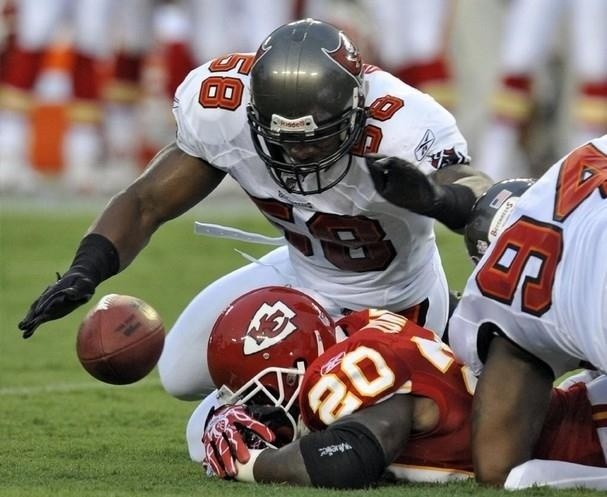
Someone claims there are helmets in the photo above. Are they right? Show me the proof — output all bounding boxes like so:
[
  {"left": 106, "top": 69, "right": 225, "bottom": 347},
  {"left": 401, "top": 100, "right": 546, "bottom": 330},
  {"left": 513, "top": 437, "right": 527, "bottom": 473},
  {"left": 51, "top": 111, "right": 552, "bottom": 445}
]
[
  {"left": 207, "top": 286, "right": 336, "bottom": 410},
  {"left": 246, "top": 18, "right": 367, "bottom": 144},
  {"left": 464, "top": 178, "right": 535, "bottom": 265}
]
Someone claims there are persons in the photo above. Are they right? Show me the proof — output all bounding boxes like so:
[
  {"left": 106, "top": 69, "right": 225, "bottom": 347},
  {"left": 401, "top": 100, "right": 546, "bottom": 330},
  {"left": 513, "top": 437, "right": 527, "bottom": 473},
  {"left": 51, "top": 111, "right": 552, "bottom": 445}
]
[
  {"left": 18, "top": 17, "right": 492, "bottom": 401},
  {"left": 202, "top": 286, "right": 606, "bottom": 486},
  {"left": 448, "top": 133, "right": 606, "bottom": 492}
]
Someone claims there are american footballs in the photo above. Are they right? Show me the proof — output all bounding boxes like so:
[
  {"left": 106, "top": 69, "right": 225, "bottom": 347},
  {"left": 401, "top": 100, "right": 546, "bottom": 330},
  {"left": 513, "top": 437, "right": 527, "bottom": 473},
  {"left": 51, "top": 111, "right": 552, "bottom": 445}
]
[{"left": 77, "top": 294, "right": 166, "bottom": 385}]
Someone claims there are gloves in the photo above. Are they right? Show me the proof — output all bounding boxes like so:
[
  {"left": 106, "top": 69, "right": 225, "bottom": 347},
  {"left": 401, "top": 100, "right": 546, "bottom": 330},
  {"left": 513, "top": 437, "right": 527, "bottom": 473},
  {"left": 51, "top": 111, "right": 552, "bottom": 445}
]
[
  {"left": 201, "top": 403, "right": 276, "bottom": 483},
  {"left": 365, "top": 153, "right": 457, "bottom": 222},
  {"left": 17, "top": 234, "right": 119, "bottom": 340}
]
